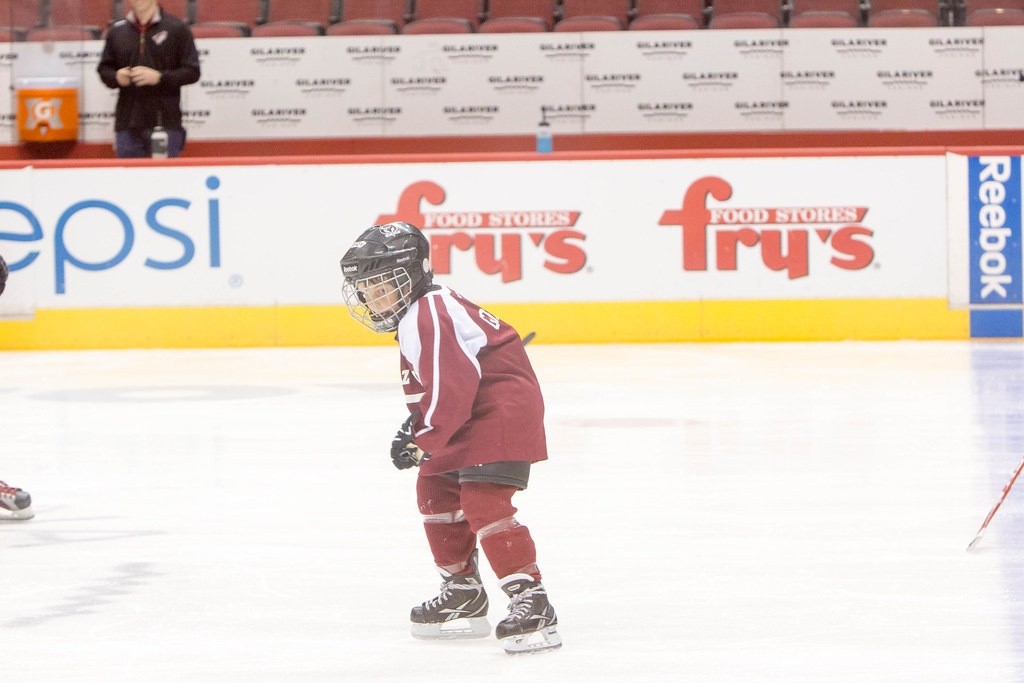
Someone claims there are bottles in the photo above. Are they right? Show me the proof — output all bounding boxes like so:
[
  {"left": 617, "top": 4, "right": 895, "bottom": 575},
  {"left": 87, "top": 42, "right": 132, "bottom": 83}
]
[
  {"left": 536, "top": 121, "right": 552, "bottom": 152},
  {"left": 150, "top": 126, "right": 169, "bottom": 159}
]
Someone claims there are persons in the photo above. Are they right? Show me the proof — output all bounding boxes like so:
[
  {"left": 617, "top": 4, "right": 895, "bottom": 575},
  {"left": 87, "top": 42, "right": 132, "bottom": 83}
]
[
  {"left": 339, "top": 219, "right": 561, "bottom": 652},
  {"left": 0, "top": 478, "right": 35, "bottom": 520},
  {"left": 96, "top": 1, "right": 201, "bottom": 158}
]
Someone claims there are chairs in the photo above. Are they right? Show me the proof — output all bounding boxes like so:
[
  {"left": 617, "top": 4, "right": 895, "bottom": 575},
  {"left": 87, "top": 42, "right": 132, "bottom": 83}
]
[{"left": 0, "top": 0, "right": 1023, "bottom": 42}]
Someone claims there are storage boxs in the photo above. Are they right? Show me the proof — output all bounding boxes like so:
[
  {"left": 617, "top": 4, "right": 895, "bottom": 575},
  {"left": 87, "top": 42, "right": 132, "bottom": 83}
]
[{"left": 15, "top": 88, "right": 79, "bottom": 142}]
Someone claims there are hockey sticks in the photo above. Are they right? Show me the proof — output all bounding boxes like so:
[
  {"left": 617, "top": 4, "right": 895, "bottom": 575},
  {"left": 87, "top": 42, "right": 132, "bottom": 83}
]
[
  {"left": 400, "top": 332, "right": 535, "bottom": 459},
  {"left": 965, "top": 454, "right": 1024, "bottom": 554}
]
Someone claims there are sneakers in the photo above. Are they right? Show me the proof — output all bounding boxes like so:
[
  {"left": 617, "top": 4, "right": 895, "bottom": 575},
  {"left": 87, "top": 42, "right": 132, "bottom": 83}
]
[
  {"left": 495, "top": 573, "right": 562, "bottom": 657},
  {"left": 409, "top": 548, "right": 492, "bottom": 640}
]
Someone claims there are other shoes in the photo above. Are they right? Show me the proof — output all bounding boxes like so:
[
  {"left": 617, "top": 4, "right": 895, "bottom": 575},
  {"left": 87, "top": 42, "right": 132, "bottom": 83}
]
[{"left": 0, "top": 481, "right": 35, "bottom": 521}]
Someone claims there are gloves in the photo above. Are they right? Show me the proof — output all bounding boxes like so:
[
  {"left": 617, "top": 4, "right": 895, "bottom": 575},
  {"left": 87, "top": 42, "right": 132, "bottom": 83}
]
[{"left": 390, "top": 416, "right": 432, "bottom": 470}]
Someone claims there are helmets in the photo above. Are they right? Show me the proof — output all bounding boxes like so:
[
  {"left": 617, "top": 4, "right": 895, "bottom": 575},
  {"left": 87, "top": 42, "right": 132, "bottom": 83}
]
[{"left": 340, "top": 221, "right": 433, "bottom": 333}]
